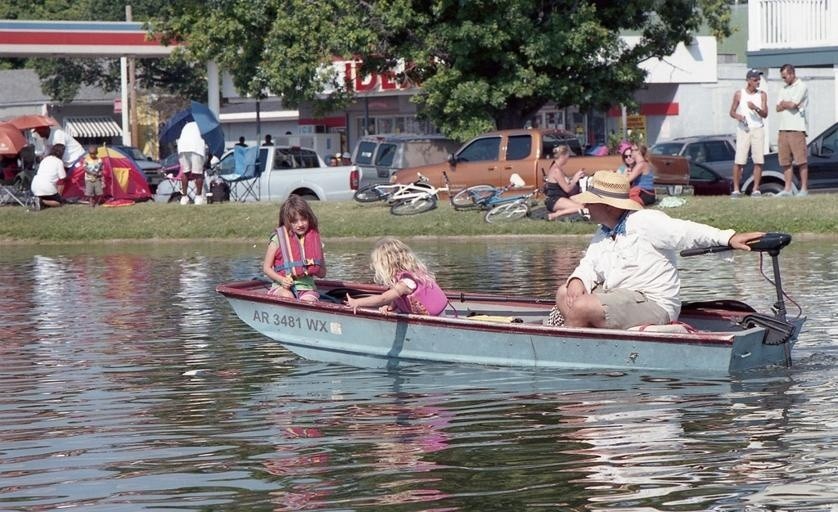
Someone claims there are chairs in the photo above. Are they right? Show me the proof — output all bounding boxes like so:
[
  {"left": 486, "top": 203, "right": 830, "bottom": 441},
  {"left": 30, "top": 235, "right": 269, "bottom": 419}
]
[
  {"left": 218, "top": 144, "right": 264, "bottom": 204},
  {"left": 159, "top": 163, "right": 197, "bottom": 199}
]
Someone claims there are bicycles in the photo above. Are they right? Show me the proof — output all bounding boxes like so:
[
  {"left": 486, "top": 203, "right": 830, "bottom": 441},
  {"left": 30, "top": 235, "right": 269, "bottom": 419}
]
[
  {"left": 352, "top": 170, "right": 454, "bottom": 216},
  {"left": 452, "top": 168, "right": 551, "bottom": 225}
]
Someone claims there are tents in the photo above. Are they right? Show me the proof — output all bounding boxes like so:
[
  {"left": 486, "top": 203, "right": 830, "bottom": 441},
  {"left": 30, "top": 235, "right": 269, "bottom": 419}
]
[{"left": 61, "top": 142, "right": 154, "bottom": 206}]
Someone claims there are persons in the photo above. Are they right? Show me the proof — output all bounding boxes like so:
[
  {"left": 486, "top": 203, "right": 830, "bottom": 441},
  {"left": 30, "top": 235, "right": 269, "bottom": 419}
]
[
  {"left": 555, "top": 169, "right": 767, "bottom": 328},
  {"left": 176, "top": 121, "right": 208, "bottom": 206},
  {"left": 728, "top": 68, "right": 768, "bottom": 198},
  {"left": 625, "top": 141, "right": 656, "bottom": 206},
  {"left": 82, "top": 145, "right": 106, "bottom": 208},
  {"left": 542, "top": 145, "right": 587, "bottom": 220},
  {"left": 341, "top": 236, "right": 450, "bottom": 318},
  {"left": 615, "top": 147, "right": 636, "bottom": 177},
  {"left": 262, "top": 194, "right": 327, "bottom": 302},
  {"left": 774, "top": 63, "right": 809, "bottom": 198},
  {"left": 234, "top": 136, "right": 248, "bottom": 147},
  {"left": 328, "top": 152, "right": 353, "bottom": 166},
  {"left": 261, "top": 134, "right": 274, "bottom": 146},
  {"left": 0, "top": 120, "right": 84, "bottom": 211}
]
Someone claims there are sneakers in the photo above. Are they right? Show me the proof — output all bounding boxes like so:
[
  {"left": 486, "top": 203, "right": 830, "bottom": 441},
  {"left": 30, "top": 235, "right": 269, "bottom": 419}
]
[
  {"left": 34, "top": 197, "right": 41, "bottom": 211},
  {"left": 180, "top": 196, "right": 189, "bottom": 205},
  {"left": 797, "top": 190, "right": 807, "bottom": 198},
  {"left": 731, "top": 192, "right": 741, "bottom": 199},
  {"left": 751, "top": 190, "right": 761, "bottom": 198},
  {"left": 194, "top": 195, "right": 203, "bottom": 205},
  {"left": 775, "top": 190, "right": 793, "bottom": 196}
]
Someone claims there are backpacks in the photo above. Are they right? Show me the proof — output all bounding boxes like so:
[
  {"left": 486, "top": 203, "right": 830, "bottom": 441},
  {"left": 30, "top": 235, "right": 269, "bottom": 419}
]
[{"left": 209, "top": 179, "right": 229, "bottom": 203}]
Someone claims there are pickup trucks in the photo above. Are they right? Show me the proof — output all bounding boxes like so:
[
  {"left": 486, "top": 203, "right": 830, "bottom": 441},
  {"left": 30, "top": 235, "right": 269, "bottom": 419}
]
[
  {"left": 646, "top": 134, "right": 735, "bottom": 179},
  {"left": 388, "top": 127, "right": 690, "bottom": 201},
  {"left": 155, "top": 146, "right": 363, "bottom": 202}
]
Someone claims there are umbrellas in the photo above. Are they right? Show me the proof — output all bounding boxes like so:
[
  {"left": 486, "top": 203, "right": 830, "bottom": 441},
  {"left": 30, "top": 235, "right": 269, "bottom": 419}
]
[
  {"left": 157, "top": 108, "right": 195, "bottom": 145},
  {"left": 6, "top": 114, "right": 56, "bottom": 129},
  {"left": 190, "top": 99, "right": 224, "bottom": 159}
]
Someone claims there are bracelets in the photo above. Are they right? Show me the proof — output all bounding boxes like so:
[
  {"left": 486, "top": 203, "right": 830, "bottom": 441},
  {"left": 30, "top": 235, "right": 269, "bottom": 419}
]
[{"left": 755, "top": 107, "right": 761, "bottom": 113}]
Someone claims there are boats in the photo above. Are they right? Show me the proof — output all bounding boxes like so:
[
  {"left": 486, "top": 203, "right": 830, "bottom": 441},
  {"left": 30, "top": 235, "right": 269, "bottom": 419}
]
[{"left": 216, "top": 232, "right": 808, "bottom": 377}]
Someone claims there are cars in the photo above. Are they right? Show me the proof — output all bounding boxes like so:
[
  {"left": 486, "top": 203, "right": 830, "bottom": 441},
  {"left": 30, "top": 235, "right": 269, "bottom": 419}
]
[
  {"left": 112, "top": 145, "right": 164, "bottom": 185},
  {"left": 740, "top": 121, "right": 838, "bottom": 195}
]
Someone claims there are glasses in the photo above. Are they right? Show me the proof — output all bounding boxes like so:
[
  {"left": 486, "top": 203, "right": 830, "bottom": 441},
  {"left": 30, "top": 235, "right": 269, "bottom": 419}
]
[{"left": 624, "top": 155, "right": 630, "bottom": 158}]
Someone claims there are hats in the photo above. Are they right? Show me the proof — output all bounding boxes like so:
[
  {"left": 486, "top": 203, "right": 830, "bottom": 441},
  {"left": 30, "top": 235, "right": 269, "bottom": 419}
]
[
  {"left": 569, "top": 170, "right": 644, "bottom": 210},
  {"left": 746, "top": 69, "right": 763, "bottom": 78}
]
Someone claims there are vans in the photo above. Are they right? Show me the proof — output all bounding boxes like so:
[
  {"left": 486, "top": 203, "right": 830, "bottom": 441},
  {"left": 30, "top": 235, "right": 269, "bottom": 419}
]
[{"left": 351, "top": 134, "right": 460, "bottom": 185}]
